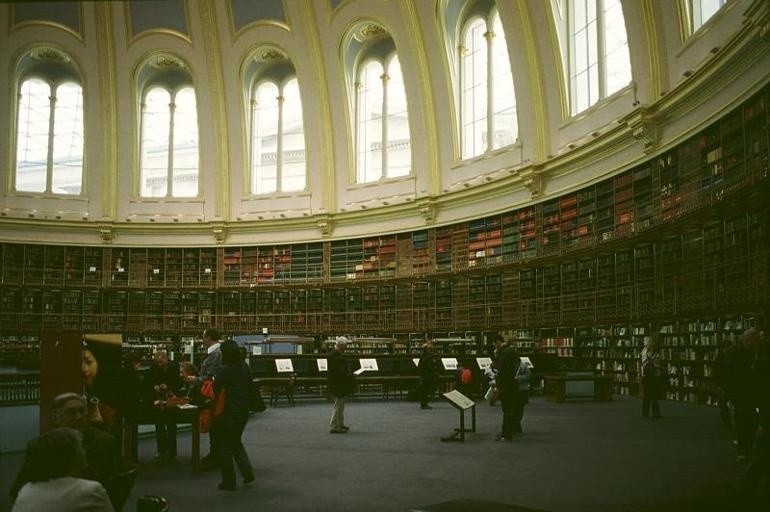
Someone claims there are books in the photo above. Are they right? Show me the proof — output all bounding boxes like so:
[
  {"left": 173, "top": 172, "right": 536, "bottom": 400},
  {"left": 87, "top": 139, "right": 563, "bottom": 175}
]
[
  {"left": 330, "top": 229, "right": 429, "bottom": 356},
  {"left": 660, "top": 91, "right": 769, "bottom": 407},
  {"left": 3, "top": 243, "right": 102, "bottom": 355},
  {"left": 109, "top": 247, "right": 216, "bottom": 368},
  {"left": 561, "top": 160, "right": 658, "bottom": 398},
  {"left": 223, "top": 243, "right": 324, "bottom": 355},
  {"left": 435, "top": 197, "right": 560, "bottom": 355}
]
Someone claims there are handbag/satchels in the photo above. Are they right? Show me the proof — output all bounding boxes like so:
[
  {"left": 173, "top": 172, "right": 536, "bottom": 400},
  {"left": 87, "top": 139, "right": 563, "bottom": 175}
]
[{"left": 249, "top": 383, "right": 267, "bottom": 412}]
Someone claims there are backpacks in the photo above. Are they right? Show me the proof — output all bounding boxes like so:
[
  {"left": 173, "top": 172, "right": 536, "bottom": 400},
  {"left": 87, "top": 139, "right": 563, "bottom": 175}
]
[{"left": 642, "top": 352, "right": 655, "bottom": 376}]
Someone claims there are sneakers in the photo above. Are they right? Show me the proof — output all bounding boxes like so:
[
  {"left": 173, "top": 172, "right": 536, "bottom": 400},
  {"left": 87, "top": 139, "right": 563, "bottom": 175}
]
[
  {"left": 495, "top": 426, "right": 522, "bottom": 441},
  {"left": 219, "top": 482, "right": 236, "bottom": 490},
  {"left": 721, "top": 418, "right": 753, "bottom": 464},
  {"left": 420, "top": 406, "right": 432, "bottom": 409},
  {"left": 640, "top": 411, "right": 664, "bottom": 419},
  {"left": 329, "top": 425, "right": 349, "bottom": 433},
  {"left": 155, "top": 448, "right": 178, "bottom": 460},
  {"left": 243, "top": 478, "right": 255, "bottom": 483}
]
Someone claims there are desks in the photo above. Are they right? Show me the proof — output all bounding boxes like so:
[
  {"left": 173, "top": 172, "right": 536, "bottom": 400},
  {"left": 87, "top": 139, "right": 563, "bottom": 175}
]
[{"left": 157, "top": 399, "right": 201, "bottom": 461}]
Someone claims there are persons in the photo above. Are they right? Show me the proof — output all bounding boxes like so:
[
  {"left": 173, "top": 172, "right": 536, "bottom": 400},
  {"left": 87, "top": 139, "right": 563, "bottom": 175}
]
[
  {"left": 637, "top": 333, "right": 664, "bottom": 420},
  {"left": 485, "top": 332, "right": 533, "bottom": 444},
  {"left": 9, "top": 426, "right": 124, "bottom": 511},
  {"left": 10, "top": 390, "right": 125, "bottom": 505},
  {"left": 710, "top": 326, "right": 770, "bottom": 474},
  {"left": 124, "top": 323, "right": 268, "bottom": 491},
  {"left": 329, "top": 335, "right": 358, "bottom": 435},
  {"left": 83, "top": 337, "right": 122, "bottom": 436},
  {"left": 418, "top": 342, "right": 436, "bottom": 410}
]
